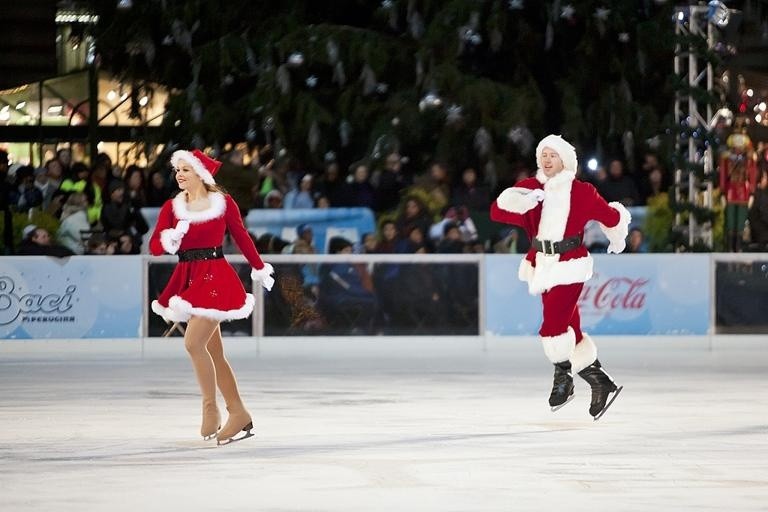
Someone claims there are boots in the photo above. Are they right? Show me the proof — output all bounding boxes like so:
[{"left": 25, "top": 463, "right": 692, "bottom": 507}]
[
  {"left": 548, "top": 359, "right": 575, "bottom": 407},
  {"left": 216, "top": 398, "right": 253, "bottom": 441},
  {"left": 578, "top": 358, "right": 618, "bottom": 417},
  {"left": 201, "top": 397, "right": 222, "bottom": 437}
]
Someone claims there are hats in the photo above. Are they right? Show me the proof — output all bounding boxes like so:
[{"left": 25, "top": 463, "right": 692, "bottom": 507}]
[
  {"left": 170, "top": 148, "right": 223, "bottom": 185},
  {"left": 536, "top": 134, "right": 579, "bottom": 175}
]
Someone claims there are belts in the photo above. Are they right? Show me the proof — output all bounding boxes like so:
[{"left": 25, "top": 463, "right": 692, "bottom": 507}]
[{"left": 531, "top": 235, "right": 581, "bottom": 254}]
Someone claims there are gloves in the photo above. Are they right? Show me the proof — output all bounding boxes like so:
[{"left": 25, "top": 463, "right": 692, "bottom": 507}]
[
  {"left": 606, "top": 239, "right": 626, "bottom": 256},
  {"left": 526, "top": 188, "right": 546, "bottom": 202},
  {"left": 169, "top": 220, "right": 190, "bottom": 241},
  {"left": 262, "top": 275, "right": 275, "bottom": 292}
]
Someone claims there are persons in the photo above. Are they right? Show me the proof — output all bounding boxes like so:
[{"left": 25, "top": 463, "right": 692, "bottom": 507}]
[
  {"left": 489, "top": 133, "right": 633, "bottom": 416},
  {"left": 145, "top": 148, "right": 275, "bottom": 442},
  {"left": 198, "top": 104, "right": 539, "bottom": 335},
  {"left": 3, "top": 149, "right": 187, "bottom": 336},
  {"left": 557, "top": 103, "right": 765, "bottom": 327}
]
